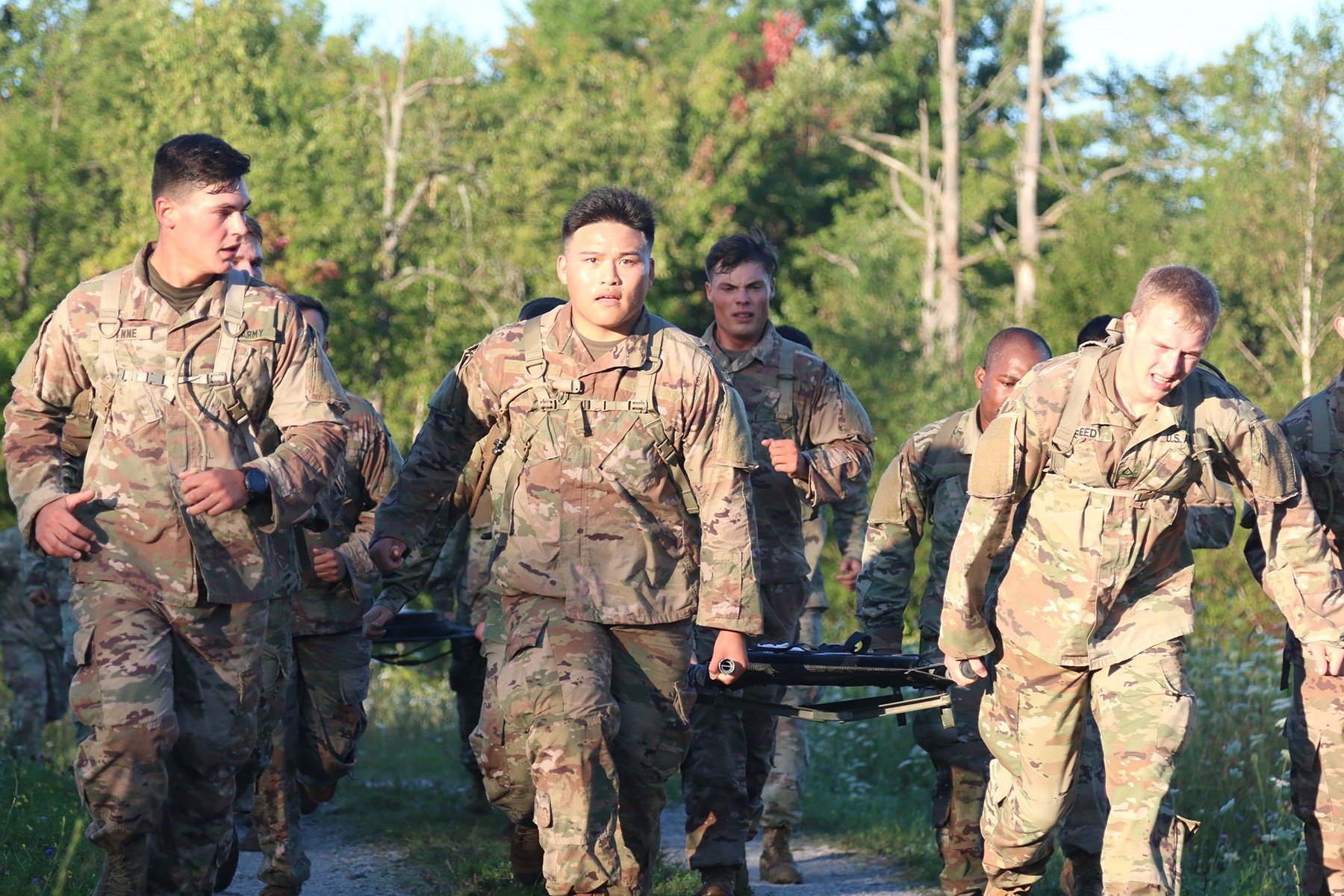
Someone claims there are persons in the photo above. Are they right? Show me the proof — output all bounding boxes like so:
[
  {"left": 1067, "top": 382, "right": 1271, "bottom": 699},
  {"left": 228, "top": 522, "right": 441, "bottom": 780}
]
[
  {"left": 677, "top": 232, "right": 873, "bottom": 895},
  {"left": 938, "top": 265, "right": 1344, "bottom": 895},
  {"left": 2, "top": 135, "right": 353, "bottom": 895},
  {"left": 368, "top": 185, "right": 764, "bottom": 896},
  {"left": 2, "top": 130, "right": 1344, "bottom": 896}
]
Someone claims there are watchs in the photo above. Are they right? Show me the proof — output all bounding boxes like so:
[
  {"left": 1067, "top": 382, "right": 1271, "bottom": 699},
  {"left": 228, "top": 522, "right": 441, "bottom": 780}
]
[{"left": 238, "top": 466, "right": 269, "bottom": 505}]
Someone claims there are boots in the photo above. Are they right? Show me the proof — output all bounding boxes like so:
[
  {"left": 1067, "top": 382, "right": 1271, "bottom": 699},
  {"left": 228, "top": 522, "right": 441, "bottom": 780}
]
[{"left": 759, "top": 827, "right": 803, "bottom": 884}]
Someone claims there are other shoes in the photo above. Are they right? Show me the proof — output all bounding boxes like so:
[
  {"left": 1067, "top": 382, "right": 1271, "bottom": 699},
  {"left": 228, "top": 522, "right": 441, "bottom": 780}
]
[
  {"left": 1059, "top": 850, "right": 1102, "bottom": 896},
  {"left": 299, "top": 784, "right": 319, "bottom": 815},
  {"left": 231, "top": 811, "right": 262, "bottom": 852},
  {"left": 511, "top": 822, "right": 544, "bottom": 884},
  {"left": 93, "top": 836, "right": 148, "bottom": 896},
  {"left": 983, "top": 880, "right": 1032, "bottom": 896},
  {"left": 259, "top": 884, "right": 302, "bottom": 896},
  {"left": 213, "top": 819, "right": 241, "bottom": 893},
  {"left": 693, "top": 865, "right": 749, "bottom": 896}
]
